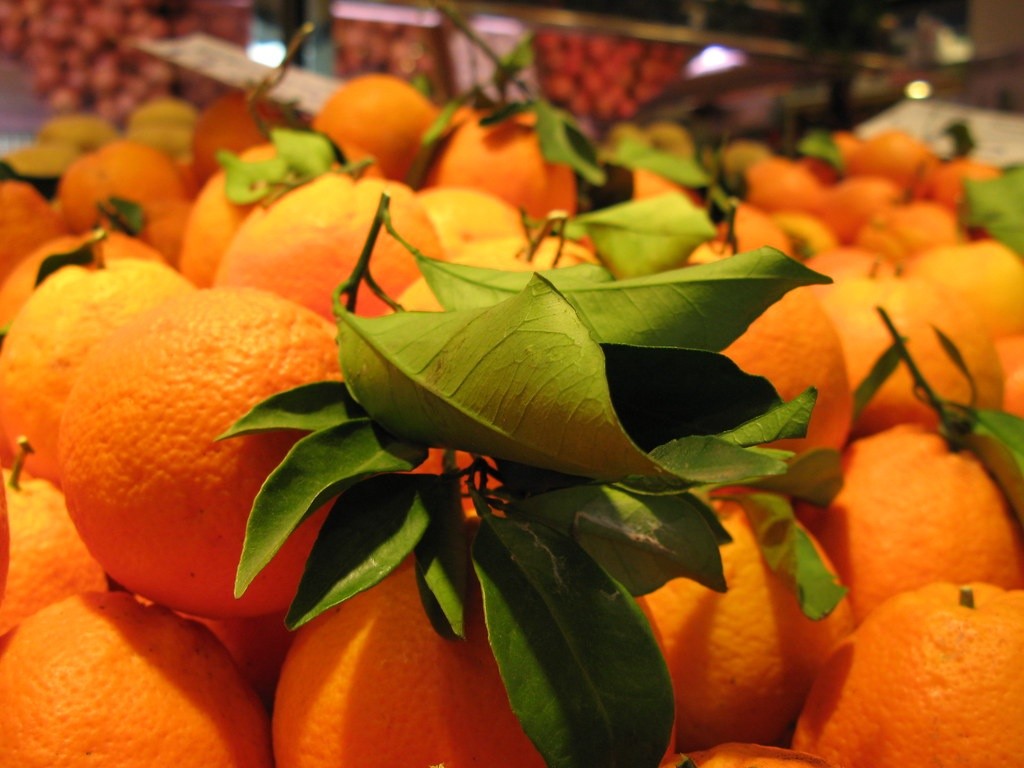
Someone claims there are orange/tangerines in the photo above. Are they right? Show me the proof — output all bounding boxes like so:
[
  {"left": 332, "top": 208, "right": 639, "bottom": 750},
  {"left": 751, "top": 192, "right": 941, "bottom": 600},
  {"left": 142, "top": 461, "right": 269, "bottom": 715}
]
[{"left": 0, "top": 73, "right": 1024, "bottom": 768}]
